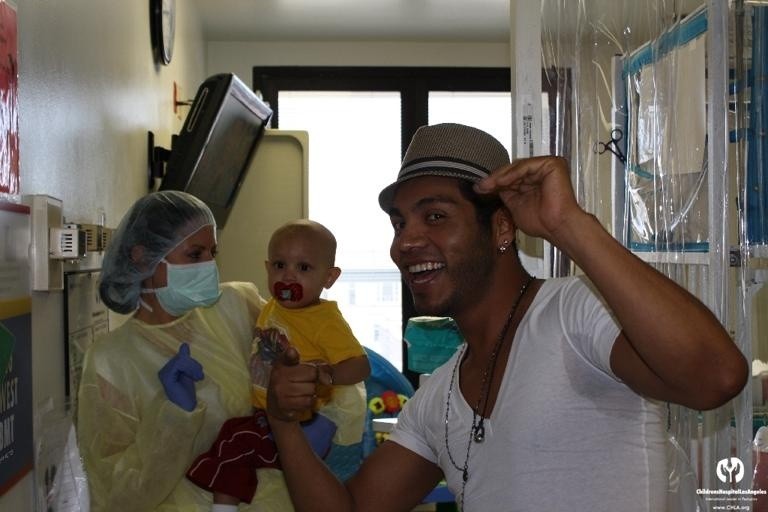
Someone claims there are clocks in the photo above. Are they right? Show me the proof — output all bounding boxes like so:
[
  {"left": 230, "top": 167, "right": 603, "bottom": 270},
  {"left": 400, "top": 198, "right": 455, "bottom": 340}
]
[{"left": 149, "top": 0, "right": 178, "bottom": 64}]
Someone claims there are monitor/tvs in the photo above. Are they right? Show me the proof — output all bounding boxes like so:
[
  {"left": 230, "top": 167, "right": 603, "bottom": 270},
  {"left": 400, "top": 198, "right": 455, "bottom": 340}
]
[{"left": 158, "top": 71, "right": 273, "bottom": 230}]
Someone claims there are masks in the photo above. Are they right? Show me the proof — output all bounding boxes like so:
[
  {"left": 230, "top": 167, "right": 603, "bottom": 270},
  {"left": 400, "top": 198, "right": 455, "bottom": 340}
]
[{"left": 141, "top": 258, "right": 223, "bottom": 319}]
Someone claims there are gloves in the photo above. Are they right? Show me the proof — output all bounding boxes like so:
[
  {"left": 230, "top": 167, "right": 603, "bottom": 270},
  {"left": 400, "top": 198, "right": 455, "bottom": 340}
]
[{"left": 157, "top": 342, "right": 205, "bottom": 412}]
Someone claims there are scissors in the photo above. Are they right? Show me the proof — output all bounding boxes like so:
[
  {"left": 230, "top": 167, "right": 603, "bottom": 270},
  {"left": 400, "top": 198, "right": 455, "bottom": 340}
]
[{"left": 592, "top": 128, "right": 627, "bottom": 166}]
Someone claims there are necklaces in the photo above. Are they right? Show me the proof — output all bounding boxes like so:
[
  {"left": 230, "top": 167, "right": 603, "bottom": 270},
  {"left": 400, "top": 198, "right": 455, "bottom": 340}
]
[{"left": 438, "top": 276, "right": 539, "bottom": 508}]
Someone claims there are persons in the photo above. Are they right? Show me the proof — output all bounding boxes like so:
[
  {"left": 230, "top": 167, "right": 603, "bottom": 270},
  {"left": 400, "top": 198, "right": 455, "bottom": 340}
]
[
  {"left": 266, "top": 119, "right": 751, "bottom": 510},
  {"left": 73, "top": 189, "right": 370, "bottom": 511},
  {"left": 185, "top": 216, "right": 375, "bottom": 511}
]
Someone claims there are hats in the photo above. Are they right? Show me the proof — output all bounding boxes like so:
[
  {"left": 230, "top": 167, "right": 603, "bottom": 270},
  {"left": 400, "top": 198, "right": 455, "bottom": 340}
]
[{"left": 378, "top": 122, "right": 512, "bottom": 215}]
